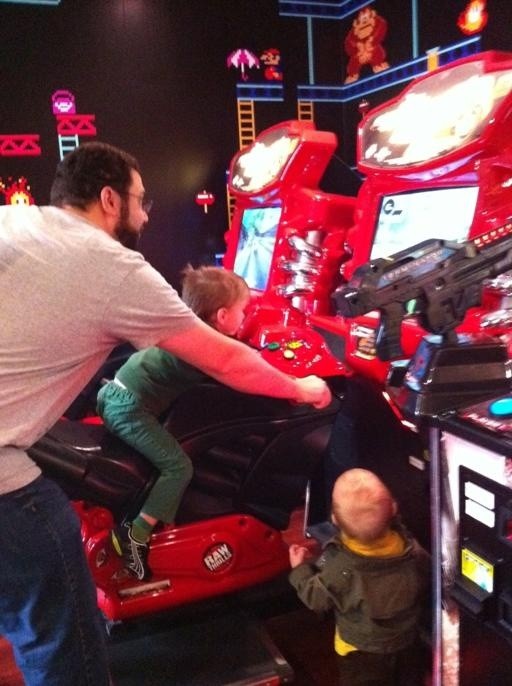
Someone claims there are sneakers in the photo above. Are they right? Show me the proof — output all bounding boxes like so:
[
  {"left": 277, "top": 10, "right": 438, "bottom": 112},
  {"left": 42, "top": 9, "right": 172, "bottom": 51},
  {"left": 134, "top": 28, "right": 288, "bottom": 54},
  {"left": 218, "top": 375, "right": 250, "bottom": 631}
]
[{"left": 109, "top": 522, "right": 153, "bottom": 582}]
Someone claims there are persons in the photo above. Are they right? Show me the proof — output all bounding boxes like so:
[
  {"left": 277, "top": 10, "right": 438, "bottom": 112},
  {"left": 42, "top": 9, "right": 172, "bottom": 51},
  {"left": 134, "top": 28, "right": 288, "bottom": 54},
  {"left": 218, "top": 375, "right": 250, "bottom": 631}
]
[
  {"left": 94, "top": 260, "right": 251, "bottom": 583},
  {"left": 285, "top": 465, "right": 435, "bottom": 686},
  {"left": 0, "top": 140, "right": 333, "bottom": 685}
]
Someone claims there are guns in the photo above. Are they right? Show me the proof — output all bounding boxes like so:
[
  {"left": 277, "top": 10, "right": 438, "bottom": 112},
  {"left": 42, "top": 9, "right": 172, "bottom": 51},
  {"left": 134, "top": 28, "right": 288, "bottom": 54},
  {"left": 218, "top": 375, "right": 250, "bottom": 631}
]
[{"left": 333, "top": 238, "right": 511, "bottom": 361}]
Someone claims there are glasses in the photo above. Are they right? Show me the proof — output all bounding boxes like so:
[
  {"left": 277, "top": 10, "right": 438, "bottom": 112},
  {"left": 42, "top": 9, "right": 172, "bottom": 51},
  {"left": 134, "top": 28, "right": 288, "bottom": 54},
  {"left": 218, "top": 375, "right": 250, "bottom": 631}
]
[{"left": 115, "top": 190, "right": 155, "bottom": 215}]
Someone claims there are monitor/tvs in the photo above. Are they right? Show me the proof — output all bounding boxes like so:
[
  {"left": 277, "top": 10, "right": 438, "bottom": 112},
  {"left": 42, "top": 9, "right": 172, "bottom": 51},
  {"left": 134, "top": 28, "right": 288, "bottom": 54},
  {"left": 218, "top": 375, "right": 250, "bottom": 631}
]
[
  {"left": 368, "top": 187, "right": 477, "bottom": 260},
  {"left": 233, "top": 208, "right": 283, "bottom": 291}
]
